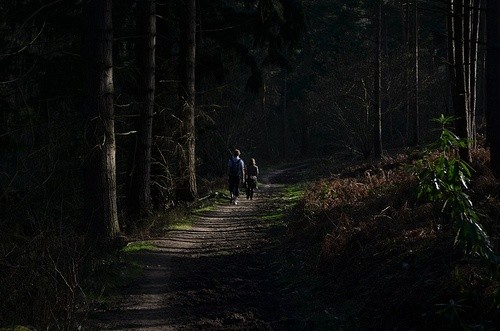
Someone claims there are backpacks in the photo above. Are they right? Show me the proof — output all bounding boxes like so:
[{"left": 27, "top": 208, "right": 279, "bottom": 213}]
[{"left": 230, "top": 158, "right": 242, "bottom": 177}]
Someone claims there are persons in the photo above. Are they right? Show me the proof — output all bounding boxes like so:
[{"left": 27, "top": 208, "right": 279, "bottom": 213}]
[
  {"left": 245, "top": 157, "right": 259, "bottom": 201},
  {"left": 227, "top": 149, "right": 245, "bottom": 204}
]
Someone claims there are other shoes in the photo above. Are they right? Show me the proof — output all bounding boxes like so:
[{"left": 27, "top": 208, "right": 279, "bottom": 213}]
[
  {"left": 246, "top": 195, "right": 252, "bottom": 200},
  {"left": 232, "top": 198, "right": 238, "bottom": 205}
]
